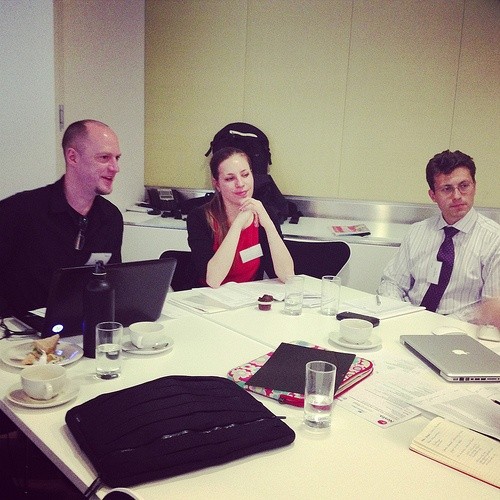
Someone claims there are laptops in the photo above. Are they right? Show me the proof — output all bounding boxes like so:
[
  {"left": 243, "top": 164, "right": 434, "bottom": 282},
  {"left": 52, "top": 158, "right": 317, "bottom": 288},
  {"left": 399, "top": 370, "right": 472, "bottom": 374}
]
[
  {"left": 399, "top": 334, "right": 500, "bottom": 382},
  {"left": 12, "top": 258, "right": 178, "bottom": 337}
]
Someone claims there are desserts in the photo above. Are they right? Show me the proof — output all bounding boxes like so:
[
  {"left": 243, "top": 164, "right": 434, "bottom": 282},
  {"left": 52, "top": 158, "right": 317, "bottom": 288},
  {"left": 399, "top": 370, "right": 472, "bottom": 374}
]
[{"left": 258, "top": 294, "right": 273, "bottom": 311}]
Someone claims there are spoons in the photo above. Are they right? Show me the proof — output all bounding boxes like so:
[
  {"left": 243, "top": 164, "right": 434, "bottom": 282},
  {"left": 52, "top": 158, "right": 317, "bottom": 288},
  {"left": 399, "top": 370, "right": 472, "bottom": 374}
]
[{"left": 124, "top": 343, "right": 169, "bottom": 352}]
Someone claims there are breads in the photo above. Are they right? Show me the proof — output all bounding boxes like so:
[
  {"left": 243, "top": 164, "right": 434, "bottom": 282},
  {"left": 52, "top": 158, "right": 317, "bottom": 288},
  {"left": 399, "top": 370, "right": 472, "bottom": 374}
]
[{"left": 21, "top": 333, "right": 60, "bottom": 366}]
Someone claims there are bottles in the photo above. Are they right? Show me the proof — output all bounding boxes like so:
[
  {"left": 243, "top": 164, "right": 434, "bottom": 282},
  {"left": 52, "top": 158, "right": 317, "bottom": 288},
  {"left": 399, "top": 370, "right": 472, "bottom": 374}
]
[{"left": 83, "top": 261, "right": 116, "bottom": 359}]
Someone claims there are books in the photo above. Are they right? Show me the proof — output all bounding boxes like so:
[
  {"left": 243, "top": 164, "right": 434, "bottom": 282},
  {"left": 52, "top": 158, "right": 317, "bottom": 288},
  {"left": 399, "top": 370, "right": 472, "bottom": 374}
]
[{"left": 409, "top": 416, "right": 500, "bottom": 490}]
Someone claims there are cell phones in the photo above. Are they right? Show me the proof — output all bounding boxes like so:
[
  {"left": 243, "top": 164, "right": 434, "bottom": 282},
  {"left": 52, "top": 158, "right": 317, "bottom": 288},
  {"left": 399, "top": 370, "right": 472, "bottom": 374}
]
[{"left": 337, "top": 312, "right": 379, "bottom": 328}]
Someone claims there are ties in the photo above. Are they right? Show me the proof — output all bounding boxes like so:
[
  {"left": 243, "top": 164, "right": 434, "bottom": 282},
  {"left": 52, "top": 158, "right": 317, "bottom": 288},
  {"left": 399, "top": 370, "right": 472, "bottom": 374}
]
[{"left": 419, "top": 226, "right": 460, "bottom": 314}]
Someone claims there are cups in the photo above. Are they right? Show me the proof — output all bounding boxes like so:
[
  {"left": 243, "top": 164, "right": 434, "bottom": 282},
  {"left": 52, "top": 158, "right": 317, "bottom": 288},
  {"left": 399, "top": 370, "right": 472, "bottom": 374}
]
[
  {"left": 340, "top": 317, "right": 374, "bottom": 344},
  {"left": 320, "top": 275, "right": 342, "bottom": 316},
  {"left": 284, "top": 275, "right": 305, "bottom": 315},
  {"left": 303, "top": 360, "right": 337, "bottom": 434},
  {"left": 95, "top": 321, "right": 123, "bottom": 380},
  {"left": 20, "top": 363, "right": 67, "bottom": 401},
  {"left": 129, "top": 321, "right": 164, "bottom": 349}
]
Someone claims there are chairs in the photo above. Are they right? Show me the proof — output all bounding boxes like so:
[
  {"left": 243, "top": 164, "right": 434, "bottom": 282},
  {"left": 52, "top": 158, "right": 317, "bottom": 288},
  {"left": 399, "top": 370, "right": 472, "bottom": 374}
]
[{"left": 261, "top": 238, "right": 350, "bottom": 282}]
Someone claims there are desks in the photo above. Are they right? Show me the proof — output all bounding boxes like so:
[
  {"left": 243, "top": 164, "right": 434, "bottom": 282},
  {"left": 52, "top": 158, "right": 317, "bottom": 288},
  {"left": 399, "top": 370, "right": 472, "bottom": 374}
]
[
  {"left": 0, "top": 273, "right": 500, "bottom": 500},
  {"left": 121, "top": 206, "right": 411, "bottom": 297}
]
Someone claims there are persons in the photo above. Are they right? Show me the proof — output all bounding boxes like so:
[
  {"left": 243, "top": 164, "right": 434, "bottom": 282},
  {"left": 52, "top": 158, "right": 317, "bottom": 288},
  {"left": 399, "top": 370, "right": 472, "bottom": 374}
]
[
  {"left": 0, "top": 119, "right": 124, "bottom": 318},
  {"left": 187, "top": 147, "right": 294, "bottom": 289},
  {"left": 376, "top": 150, "right": 500, "bottom": 329}
]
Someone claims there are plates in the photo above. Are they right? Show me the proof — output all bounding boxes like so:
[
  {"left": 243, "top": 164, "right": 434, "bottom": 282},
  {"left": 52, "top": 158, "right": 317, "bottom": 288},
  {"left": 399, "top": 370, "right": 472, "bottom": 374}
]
[
  {"left": 0, "top": 341, "right": 84, "bottom": 368},
  {"left": 6, "top": 379, "right": 81, "bottom": 409},
  {"left": 122, "top": 334, "right": 173, "bottom": 355},
  {"left": 327, "top": 329, "right": 384, "bottom": 350}
]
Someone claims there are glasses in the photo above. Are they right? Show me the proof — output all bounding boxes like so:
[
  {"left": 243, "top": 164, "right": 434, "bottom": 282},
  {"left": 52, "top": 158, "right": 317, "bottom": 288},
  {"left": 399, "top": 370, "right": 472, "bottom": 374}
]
[{"left": 435, "top": 181, "right": 477, "bottom": 197}]
